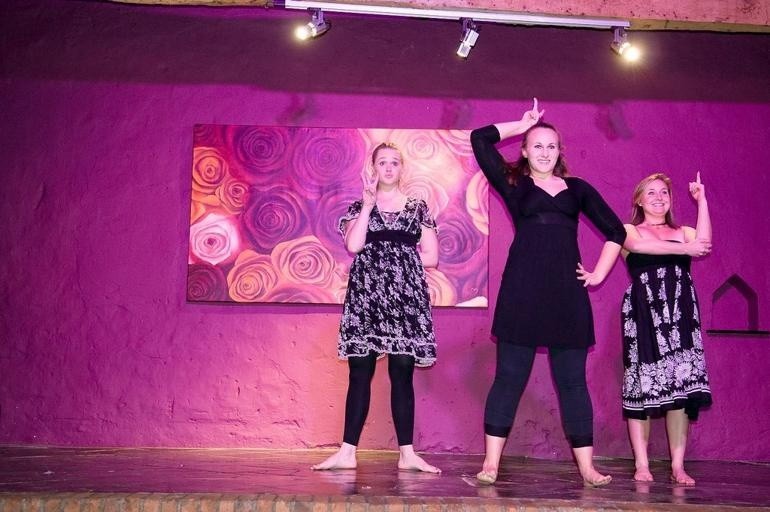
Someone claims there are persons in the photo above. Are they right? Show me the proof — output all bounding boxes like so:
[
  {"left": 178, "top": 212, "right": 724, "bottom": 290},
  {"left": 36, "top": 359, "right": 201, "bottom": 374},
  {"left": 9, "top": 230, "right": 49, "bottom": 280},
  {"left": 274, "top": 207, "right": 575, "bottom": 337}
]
[
  {"left": 310, "top": 143, "right": 443, "bottom": 474},
  {"left": 620, "top": 170, "right": 712, "bottom": 484},
  {"left": 470, "top": 97, "right": 626, "bottom": 489}
]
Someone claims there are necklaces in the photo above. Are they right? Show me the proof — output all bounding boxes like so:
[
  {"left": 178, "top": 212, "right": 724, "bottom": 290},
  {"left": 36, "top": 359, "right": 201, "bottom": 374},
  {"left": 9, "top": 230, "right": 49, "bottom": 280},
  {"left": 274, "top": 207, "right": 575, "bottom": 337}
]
[{"left": 645, "top": 223, "right": 666, "bottom": 226}]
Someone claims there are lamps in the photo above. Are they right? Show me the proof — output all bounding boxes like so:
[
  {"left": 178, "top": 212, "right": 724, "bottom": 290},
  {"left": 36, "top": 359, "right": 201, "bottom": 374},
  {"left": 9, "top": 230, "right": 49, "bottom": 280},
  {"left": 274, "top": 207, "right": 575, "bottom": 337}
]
[
  {"left": 455, "top": 17, "right": 480, "bottom": 58},
  {"left": 298, "top": 6, "right": 332, "bottom": 39},
  {"left": 610, "top": 26, "right": 631, "bottom": 54}
]
